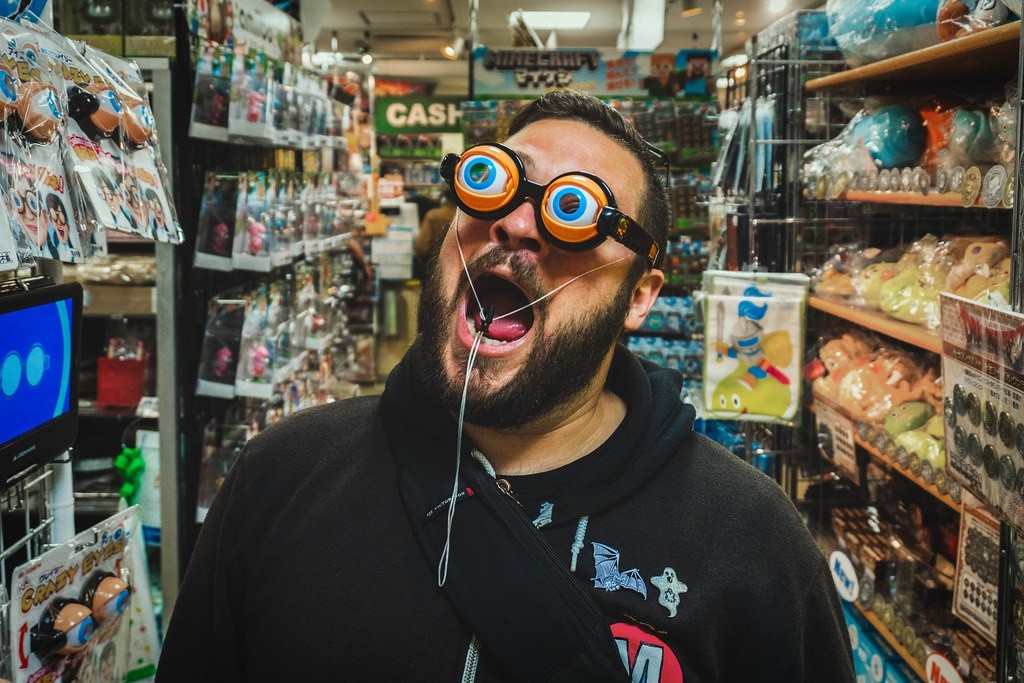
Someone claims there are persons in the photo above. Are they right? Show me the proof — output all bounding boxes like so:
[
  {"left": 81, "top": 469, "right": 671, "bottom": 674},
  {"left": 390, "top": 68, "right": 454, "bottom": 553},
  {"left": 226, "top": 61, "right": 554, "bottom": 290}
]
[
  {"left": 145, "top": 186, "right": 179, "bottom": 245},
  {"left": 112, "top": 165, "right": 161, "bottom": 241},
  {"left": 0, "top": 162, "right": 60, "bottom": 261},
  {"left": 45, "top": 194, "right": 84, "bottom": 263},
  {"left": 98, "top": 640, "right": 122, "bottom": 683},
  {"left": 61, "top": 645, "right": 99, "bottom": 683},
  {"left": 154, "top": 87, "right": 862, "bottom": 683},
  {"left": 91, "top": 165, "right": 138, "bottom": 240}
]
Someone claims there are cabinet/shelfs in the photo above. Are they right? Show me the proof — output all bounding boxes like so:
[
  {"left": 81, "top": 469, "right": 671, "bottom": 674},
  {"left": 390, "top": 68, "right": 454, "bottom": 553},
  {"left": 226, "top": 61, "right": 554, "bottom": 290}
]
[
  {"left": 375, "top": 12, "right": 833, "bottom": 509},
  {"left": 794, "top": 0, "right": 1024, "bottom": 683},
  {"left": 0, "top": 0, "right": 387, "bottom": 683}
]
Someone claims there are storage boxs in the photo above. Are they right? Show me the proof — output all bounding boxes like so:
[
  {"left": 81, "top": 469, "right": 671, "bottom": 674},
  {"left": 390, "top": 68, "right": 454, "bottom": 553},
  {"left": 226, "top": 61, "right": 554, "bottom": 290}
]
[{"left": 841, "top": 599, "right": 921, "bottom": 683}]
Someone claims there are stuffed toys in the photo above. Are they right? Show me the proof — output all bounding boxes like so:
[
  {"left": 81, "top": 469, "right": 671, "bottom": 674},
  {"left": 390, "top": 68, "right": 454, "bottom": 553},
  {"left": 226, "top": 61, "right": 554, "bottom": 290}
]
[{"left": 795, "top": 208, "right": 1015, "bottom": 450}]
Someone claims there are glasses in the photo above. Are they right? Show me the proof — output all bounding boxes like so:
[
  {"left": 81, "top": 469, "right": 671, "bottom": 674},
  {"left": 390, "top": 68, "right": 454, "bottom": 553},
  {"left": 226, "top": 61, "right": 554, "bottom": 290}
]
[
  {"left": 31, "top": 568, "right": 131, "bottom": 659},
  {"left": 71, "top": 664, "right": 93, "bottom": 683},
  {"left": 0, "top": 62, "right": 62, "bottom": 142},
  {"left": 149, "top": 199, "right": 162, "bottom": 213},
  {"left": 47, "top": 208, "right": 69, "bottom": 225},
  {"left": 96, "top": 186, "right": 116, "bottom": 200},
  {"left": 8, "top": 187, "right": 42, "bottom": 217},
  {"left": 100, "top": 654, "right": 115, "bottom": 671},
  {"left": 118, "top": 182, "right": 141, "bottom": 205},
  {"left": 439, "top": 141, "right": 659, "bottom": 269},
  {"left": 68, "top": 81, "right": 156, "bottom": 150}
]
